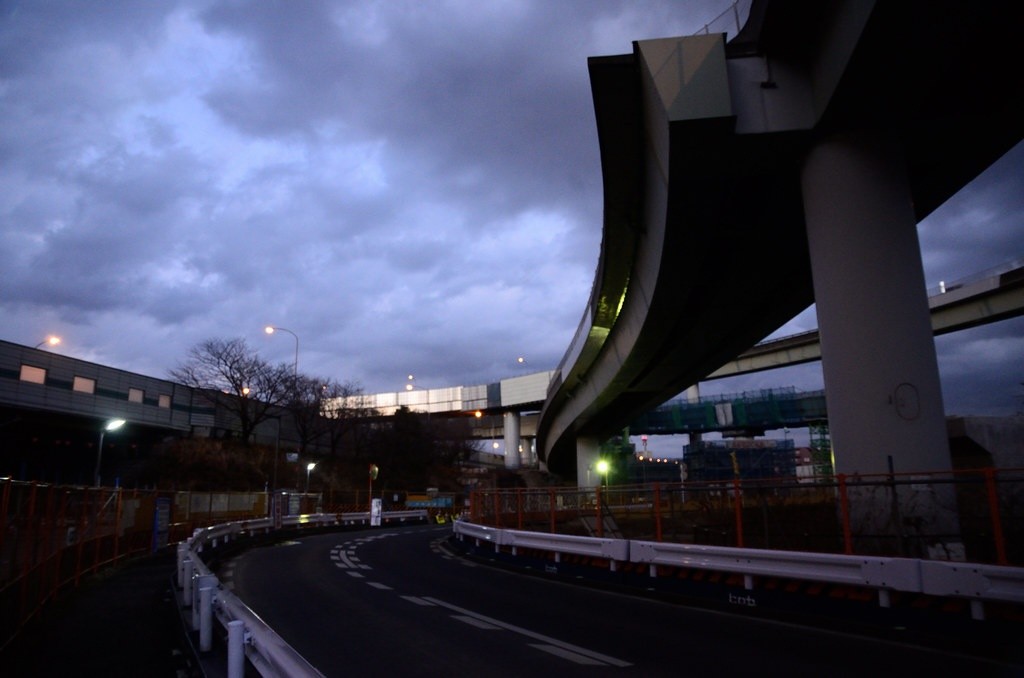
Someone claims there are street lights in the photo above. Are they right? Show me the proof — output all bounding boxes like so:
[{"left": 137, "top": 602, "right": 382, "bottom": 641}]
[
  {"left": 305, "top": 463, "right": 316, "bottom": 514},
  {"left": 409, "top": 375, "right": 417, "bottom": 391},
  {"left": 596, "top": 460, "right": 611, "bottom": 508},
  {"left": 518, "top": 357, "right": 529, "bottom": 376},
  {"left": 369, "top": 464, "right": 378, "bottom": 512},
  {"left": 94, "top": 416, "right": 126, "bottom": 486},
  {"left": 265, "top": 327, "right": 299, "bottom": 404},
  {"left": 475, "top": 411, "right": 495, "bottom": 465}
]
